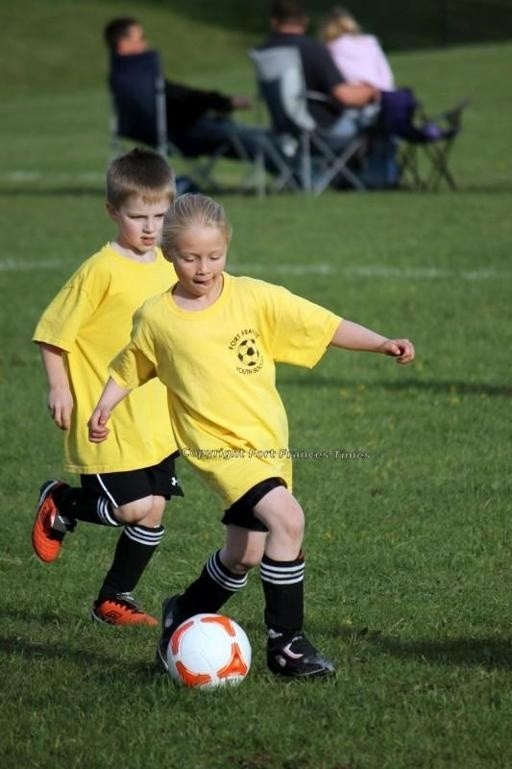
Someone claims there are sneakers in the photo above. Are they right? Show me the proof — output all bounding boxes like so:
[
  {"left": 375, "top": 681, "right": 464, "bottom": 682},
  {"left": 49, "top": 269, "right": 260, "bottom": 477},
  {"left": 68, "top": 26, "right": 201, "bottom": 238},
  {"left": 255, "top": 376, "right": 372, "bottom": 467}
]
[
  {"left": 92, "top": 592, "right": 159, "bottom": 627},
  {"left": 267, "top": 633, "right": 336, "bottom": 680},
  {"left": 32, "top": 480, "right": 77, "bottom": 563},
  {"left": 157, "top": 593, "right": 181, "bottom": 674}
]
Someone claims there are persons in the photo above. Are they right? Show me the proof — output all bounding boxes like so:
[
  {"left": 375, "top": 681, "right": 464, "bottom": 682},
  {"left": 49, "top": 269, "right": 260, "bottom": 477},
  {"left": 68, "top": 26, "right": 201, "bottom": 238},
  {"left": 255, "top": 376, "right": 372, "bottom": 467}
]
[
  {"left": 31, "top": 149, "right": 185, "bottom": 630},
  {"left": 87, "top": 194, "right": 415, "bottom": 682},
  {"left": 255, "top": 1, "right": 466, "bottom": 157},
  {"left": 104, "top": 16, "right": 302, "bottom": 187},
  {"left": 321, "top": 9, "right": 401, "bottom": 187}
]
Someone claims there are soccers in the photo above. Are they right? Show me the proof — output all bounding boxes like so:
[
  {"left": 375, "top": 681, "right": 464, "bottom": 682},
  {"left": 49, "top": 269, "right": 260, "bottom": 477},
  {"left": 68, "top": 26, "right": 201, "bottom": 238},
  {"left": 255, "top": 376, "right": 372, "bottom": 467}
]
[{"left": 167, "top": 613, "right": 252, "bottom": 691}]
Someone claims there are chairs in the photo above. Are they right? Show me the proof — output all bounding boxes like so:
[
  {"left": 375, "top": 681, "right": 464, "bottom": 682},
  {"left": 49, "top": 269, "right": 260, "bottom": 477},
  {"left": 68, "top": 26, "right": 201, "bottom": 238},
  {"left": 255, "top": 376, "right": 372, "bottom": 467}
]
[
  {"left": 373, "top": 84, "right": 466, "bottom": 189},
  {"left": 244, "top": 42, "right": 370, "bottom": 197},
  {"left": 113, "top": 46, "right": 241, "bottom": 197}
]
